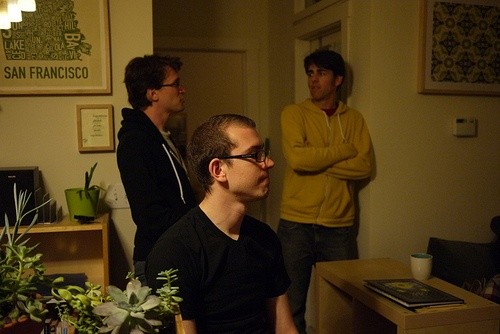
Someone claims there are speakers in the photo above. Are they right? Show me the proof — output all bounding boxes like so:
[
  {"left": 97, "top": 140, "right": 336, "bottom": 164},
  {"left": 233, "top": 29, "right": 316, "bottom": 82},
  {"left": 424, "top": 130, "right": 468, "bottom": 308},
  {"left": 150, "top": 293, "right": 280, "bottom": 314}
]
[{"left": 0, "top": 165, "right": 41, "bottom": 227}]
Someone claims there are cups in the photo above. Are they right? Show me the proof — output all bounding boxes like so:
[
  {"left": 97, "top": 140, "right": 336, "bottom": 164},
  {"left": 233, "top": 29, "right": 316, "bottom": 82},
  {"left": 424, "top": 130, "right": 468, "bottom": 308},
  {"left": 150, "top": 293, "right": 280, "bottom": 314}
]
[{"left": 410, "top": 252, "right": 434, "bottom": 282}]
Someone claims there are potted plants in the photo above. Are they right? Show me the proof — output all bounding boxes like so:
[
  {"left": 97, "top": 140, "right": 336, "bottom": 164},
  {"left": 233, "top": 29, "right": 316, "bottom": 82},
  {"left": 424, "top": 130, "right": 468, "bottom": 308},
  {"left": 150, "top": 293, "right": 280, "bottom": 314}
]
[
  {"left": 51, "top": 271, "right": 184, "bottom": 334},
  {"left": 0, "top": 181, "right": 58, "bottom": 334},
  {"left": 64, "top": 165, "right": 108, "bottom": 221}
]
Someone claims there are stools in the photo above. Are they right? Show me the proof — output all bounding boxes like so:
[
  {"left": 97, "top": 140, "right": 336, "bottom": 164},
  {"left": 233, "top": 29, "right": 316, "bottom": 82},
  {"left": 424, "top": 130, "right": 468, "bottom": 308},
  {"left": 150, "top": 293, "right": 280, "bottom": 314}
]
[{"left": 24, "top": 271, "right": 87, "bottom": 334}]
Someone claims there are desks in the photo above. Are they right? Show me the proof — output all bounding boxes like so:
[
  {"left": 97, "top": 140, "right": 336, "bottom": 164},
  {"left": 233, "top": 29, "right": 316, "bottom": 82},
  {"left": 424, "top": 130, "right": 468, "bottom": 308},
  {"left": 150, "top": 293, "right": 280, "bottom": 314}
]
[
  {"left": 1, "top": 211, "right": 111, "bottom": 308},
  {"left": 312, "top": 256, "right": 500, "bottom": 334}
]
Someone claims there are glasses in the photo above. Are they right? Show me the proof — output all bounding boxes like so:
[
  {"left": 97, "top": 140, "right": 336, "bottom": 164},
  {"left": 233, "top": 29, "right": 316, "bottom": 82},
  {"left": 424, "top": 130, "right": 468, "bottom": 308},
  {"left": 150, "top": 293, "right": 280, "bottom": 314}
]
[
  {"left": 220, "top": 137, "right": 272, "bottom": 163},
  {"left": 162, "top": 80, "right": 180, "bottom": 88}
]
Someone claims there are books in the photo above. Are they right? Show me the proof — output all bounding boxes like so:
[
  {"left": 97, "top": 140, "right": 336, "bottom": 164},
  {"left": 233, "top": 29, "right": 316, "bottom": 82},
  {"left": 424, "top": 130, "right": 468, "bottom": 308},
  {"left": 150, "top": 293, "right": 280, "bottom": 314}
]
[{"left": 361, "top": 278, "right": 464, "bottom": 309}]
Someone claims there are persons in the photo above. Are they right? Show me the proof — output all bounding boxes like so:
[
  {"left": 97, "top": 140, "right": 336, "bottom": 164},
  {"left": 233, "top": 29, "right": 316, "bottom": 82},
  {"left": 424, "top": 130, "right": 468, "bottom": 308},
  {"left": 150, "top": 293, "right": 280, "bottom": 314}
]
[
  {"left": 145, "top": 114, "right": 297, "bottom": 334},
  {"left": 277, "top": 49, "right": 376, "bottom": 334},
  {"left": 116, "top": 55, "right": 198, "bottom": 289}
]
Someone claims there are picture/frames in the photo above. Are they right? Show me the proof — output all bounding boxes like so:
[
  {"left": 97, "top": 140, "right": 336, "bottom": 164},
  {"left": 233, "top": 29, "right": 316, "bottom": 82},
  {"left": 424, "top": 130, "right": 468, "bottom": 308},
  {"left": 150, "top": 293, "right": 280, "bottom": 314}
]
[
  {"left": 76, "top": 103, "right": 114, "bottom": 152},
  {"left": 0, "top": 0, "right": 112, "bottom": 97},
  {"left": 415, "top": 0, "right": 500, "bottom": 95}
]
